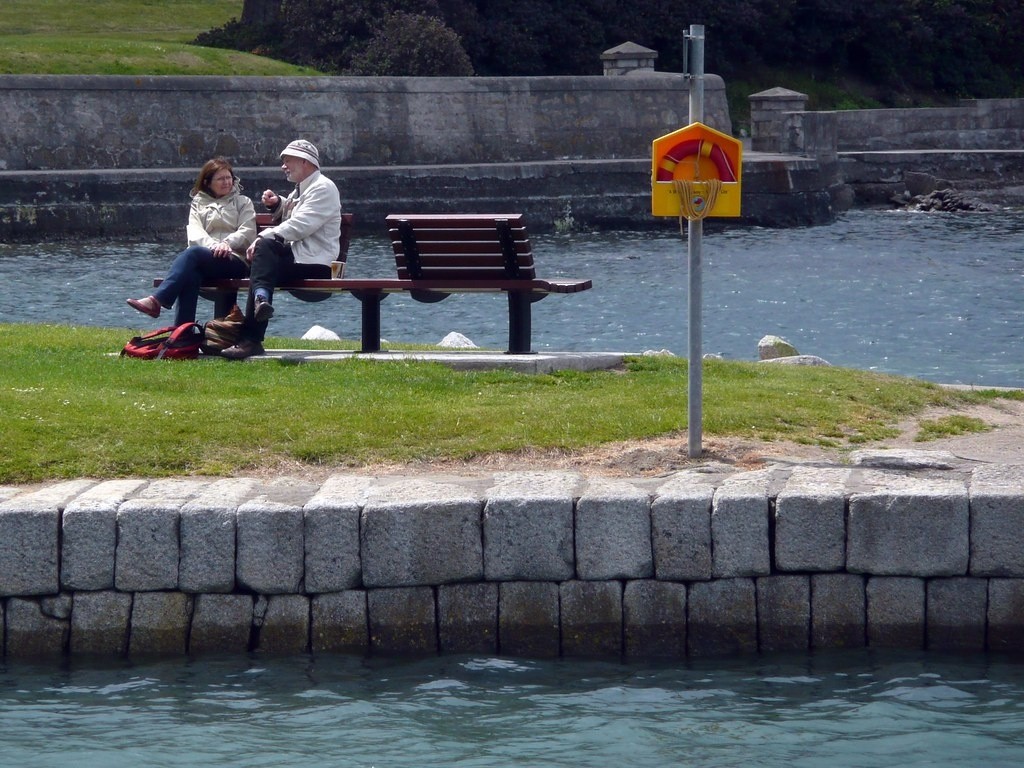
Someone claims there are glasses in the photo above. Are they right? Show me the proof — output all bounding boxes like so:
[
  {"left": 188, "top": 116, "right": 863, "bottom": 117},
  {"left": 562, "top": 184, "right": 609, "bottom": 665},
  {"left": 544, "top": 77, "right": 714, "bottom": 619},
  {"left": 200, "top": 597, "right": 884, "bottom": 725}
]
[{"left": 211, "top": 176, "right": 232, "bottom": 181}]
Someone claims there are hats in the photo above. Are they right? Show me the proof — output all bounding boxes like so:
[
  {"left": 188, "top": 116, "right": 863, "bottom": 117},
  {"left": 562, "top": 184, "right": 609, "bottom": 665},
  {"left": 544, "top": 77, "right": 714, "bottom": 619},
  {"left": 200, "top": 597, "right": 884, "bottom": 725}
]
[{"left": 279, "top": 139, "right": 320, "bottom": 169}]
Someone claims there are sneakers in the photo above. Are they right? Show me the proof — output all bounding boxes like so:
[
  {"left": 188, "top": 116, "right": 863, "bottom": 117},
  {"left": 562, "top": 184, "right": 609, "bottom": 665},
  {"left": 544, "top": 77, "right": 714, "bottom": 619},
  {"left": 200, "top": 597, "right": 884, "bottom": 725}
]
[
  {"left": 254, "top": 294, "right": 274, "bottom": 321},
  {"left": 220, "top": 336, "right": 264, "bottom": 359}
]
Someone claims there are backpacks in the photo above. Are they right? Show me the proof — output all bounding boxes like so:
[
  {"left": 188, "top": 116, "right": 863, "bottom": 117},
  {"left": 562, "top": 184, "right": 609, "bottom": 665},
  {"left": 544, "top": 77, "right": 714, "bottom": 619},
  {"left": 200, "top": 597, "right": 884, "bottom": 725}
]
[{"left": 119, "top": 320, "right": 205, "bottom": 360}]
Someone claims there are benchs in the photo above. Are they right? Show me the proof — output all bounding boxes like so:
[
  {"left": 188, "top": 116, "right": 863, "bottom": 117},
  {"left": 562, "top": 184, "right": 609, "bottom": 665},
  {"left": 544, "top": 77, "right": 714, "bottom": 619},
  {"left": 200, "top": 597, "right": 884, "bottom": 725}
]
[{"left": 153, "top": 213, "right": 594, "bottom": 355}]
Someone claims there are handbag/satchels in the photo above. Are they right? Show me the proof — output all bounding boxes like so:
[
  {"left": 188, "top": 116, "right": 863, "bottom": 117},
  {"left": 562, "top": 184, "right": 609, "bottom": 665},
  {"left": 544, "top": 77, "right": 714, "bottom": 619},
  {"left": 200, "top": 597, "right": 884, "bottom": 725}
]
[{"left": 201, "top": 304, "right": 250, "bottom": 355}]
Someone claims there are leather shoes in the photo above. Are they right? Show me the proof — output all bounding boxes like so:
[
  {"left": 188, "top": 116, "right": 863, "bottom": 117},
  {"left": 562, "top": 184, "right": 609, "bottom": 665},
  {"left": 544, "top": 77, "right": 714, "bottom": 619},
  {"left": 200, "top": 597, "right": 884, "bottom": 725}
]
[{"left": 127, "top": 296, "right": 161, "bottom": 318}]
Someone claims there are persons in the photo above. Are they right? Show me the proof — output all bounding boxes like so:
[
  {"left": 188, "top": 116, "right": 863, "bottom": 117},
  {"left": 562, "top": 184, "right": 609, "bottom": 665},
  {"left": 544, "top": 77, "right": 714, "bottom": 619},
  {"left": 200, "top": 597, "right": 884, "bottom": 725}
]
[
  {"left": 222, "top": 140, "right": 341, "bottom": 358},
  {"left": 127, "top": 158, "right": 256, "bottom": 332}
]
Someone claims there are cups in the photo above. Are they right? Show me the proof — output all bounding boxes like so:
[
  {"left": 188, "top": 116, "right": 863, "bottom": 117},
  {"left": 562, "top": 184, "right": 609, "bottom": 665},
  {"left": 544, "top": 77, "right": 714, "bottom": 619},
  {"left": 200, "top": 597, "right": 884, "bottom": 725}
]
[{"left": 331, "top": 260, "right": 345, "bottom": 279}]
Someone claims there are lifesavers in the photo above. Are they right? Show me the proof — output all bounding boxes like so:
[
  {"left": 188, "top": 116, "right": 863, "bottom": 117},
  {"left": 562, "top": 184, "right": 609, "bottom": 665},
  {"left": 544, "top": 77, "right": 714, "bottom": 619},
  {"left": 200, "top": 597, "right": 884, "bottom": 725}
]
[{"left": 656, "top": 139, "right": 735, "bottom": 183}]
